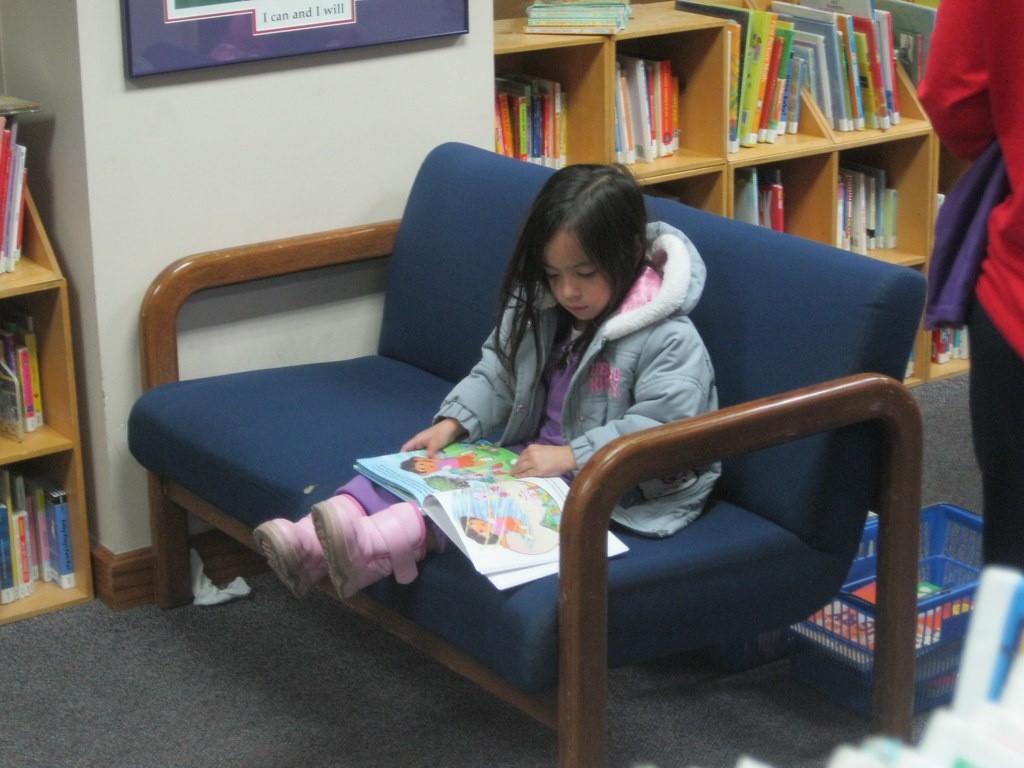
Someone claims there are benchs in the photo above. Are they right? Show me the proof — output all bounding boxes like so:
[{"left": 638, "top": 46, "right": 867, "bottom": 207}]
[{"left": 126, "top": 143, "right": 930, "bottom": 768}]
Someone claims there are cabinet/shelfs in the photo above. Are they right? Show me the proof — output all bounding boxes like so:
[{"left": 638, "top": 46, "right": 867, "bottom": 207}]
[
  {"left": 0, "top": 187, "right": 94, "bottom": 626},
  {"left": 493, "top": 1, "right": 970, "bottom": 387}
]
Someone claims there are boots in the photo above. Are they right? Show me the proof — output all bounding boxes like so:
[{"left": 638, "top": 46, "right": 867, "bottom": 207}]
[
  {"left": 252, "top": 494, "right": 367, "bottom": 600},
  {"left": 310, "top": 498, "right": 427, "bottom": 604}
]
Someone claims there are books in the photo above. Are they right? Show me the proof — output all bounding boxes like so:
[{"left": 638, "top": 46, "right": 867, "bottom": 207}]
[
  {"left": 789, "top": 579, "right": 1024, "bottom": 768},
  {"left": 493, "top": 0, "right": 964, "bottom": 378},
  {"left": 352, "top": 435, "right": 631, "bottom": 592},
  {"left": 0, "top": 113, "right": 75, "bottom": 604}
]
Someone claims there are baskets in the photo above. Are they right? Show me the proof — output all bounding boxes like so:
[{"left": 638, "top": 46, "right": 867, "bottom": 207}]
[{"left": 783, "top": 503, "right": 984, "bottom": 716}]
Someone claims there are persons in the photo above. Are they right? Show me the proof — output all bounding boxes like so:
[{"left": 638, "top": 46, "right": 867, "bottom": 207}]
[
  {"left": 917, "top": 0, "right": 1024, "bottom": 710},
  {"left": 253, "top": 163, "right": 722, "bottom": 600}
]
